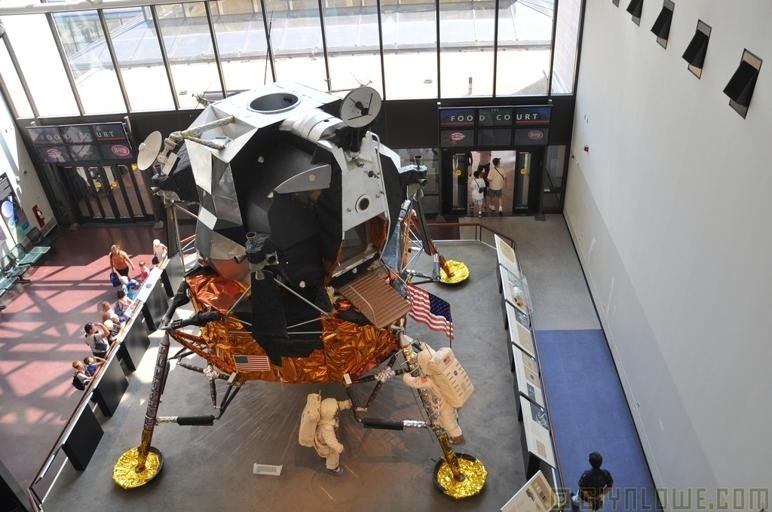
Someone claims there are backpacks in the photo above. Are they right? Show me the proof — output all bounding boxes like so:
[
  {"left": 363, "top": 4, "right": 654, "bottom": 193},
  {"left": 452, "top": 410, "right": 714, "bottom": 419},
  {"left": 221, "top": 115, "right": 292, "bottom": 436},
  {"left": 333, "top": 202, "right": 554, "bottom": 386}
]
[{"left": 72, "top": 376, "right": 84, "bottom": 390}]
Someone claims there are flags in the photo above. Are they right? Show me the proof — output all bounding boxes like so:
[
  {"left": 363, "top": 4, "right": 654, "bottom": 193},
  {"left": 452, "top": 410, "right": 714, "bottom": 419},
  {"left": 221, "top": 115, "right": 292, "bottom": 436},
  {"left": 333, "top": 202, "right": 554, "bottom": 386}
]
[{"left": 404, "top": 283, "right": 455, "bottom": 339}]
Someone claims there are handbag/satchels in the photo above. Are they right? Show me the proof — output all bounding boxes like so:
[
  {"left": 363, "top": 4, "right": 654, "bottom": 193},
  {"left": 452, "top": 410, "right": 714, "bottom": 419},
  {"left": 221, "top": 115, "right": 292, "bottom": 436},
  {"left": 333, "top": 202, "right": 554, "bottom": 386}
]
[
  {"left": 93, "top": 343, "right": 107, "bottom": 357},
  {"left": 110, "top": 272, "right": 120, "bottom": 287}
]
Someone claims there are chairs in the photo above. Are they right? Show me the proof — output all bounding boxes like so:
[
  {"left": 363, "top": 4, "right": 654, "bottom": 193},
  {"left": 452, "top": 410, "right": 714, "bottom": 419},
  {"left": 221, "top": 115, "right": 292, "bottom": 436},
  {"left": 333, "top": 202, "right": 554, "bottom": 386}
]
[{"left": 0, "top": 226, "right": 58, "bottom": 312}]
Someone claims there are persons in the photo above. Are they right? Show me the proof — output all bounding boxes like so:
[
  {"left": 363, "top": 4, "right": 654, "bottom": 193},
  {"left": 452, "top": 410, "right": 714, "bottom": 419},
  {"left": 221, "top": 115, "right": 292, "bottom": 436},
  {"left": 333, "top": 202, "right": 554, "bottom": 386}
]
[
  {"left": 109, "top": 244, "right": 136, "bottom": 280},
  {"left": 567, "top": 452, "right": 615, "bottom": 512},
  {"left": 402, "top": 340, "right": 464, "bottom": 443},
  {"left": 69, "top": 260, "right": 152, "bottom": 391},
  {"left": 152, "top": 239, "right": 168, "bottom": 263},
  {"left": 475, "top": 150, "right": 491, "bottom": 176},
  {"left": 470, "top": 170, "right": 486, "bottom": 217},
  {"left": 487, "top": 157, "right": 507, "bottom": 216},
  {"left": 312, "top": 398, "right": 354, "bottom": 474}
]
